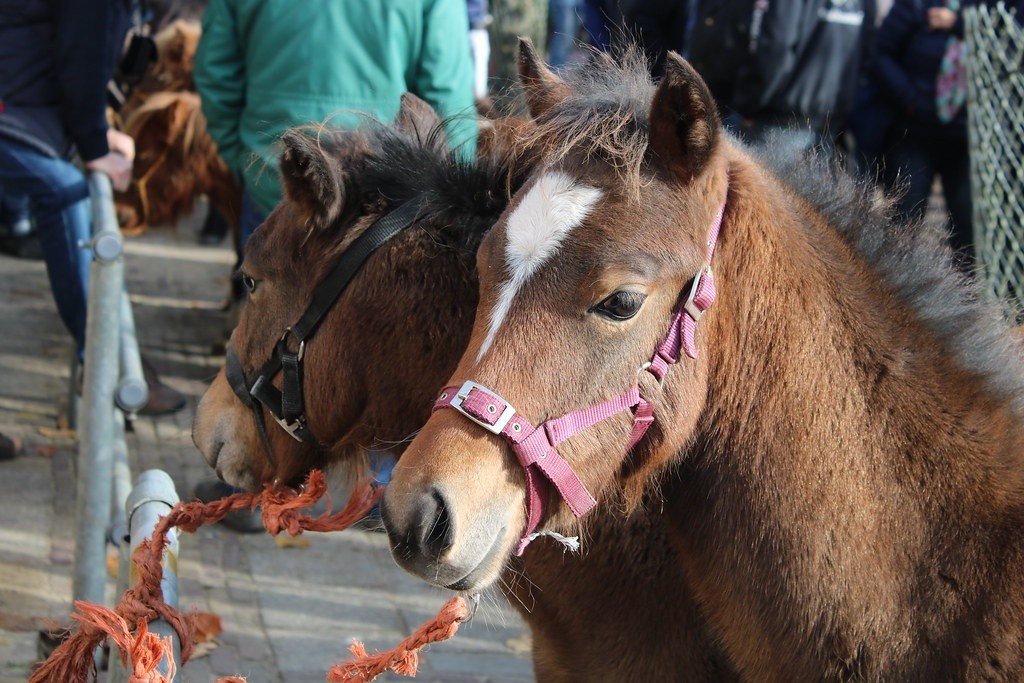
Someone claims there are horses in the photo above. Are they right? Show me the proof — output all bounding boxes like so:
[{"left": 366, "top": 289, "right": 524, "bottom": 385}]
[
  {"left": 190, "top": 91, "right": 736, "bottom": 683},
  {"left": 105, "top": 19, "right": 245, "bottom": 268},
  {"left": 379, "top": 34, "right": 1024, "bottom": 683}
]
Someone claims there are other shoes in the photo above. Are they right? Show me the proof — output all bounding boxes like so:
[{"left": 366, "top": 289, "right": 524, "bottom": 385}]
[
  {"left": 197, "top": 483, "right": 264, "bottom": 533},
  {"left": 142, "top": 361, "right": 186, "bottom": 414},
  {"left": 0, "top": 433, "right": 15, "bottom": 459}
]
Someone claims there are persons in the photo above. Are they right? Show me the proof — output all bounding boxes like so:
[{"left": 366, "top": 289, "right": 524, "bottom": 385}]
[
  {"left": 546, "top": 0, "right": 892, "bottom": 189},
  {"left": 0, "top": 0, "right": 185, "bottom": 461},
  {"left": 193, "top": 0, "right": 476, "bottom": 534},
  {"left": 468, "top": 0, "right": 493, "bottom": 102},
  {"left": 878, "top": 0, "right": 978, "bottom": 305}
]
